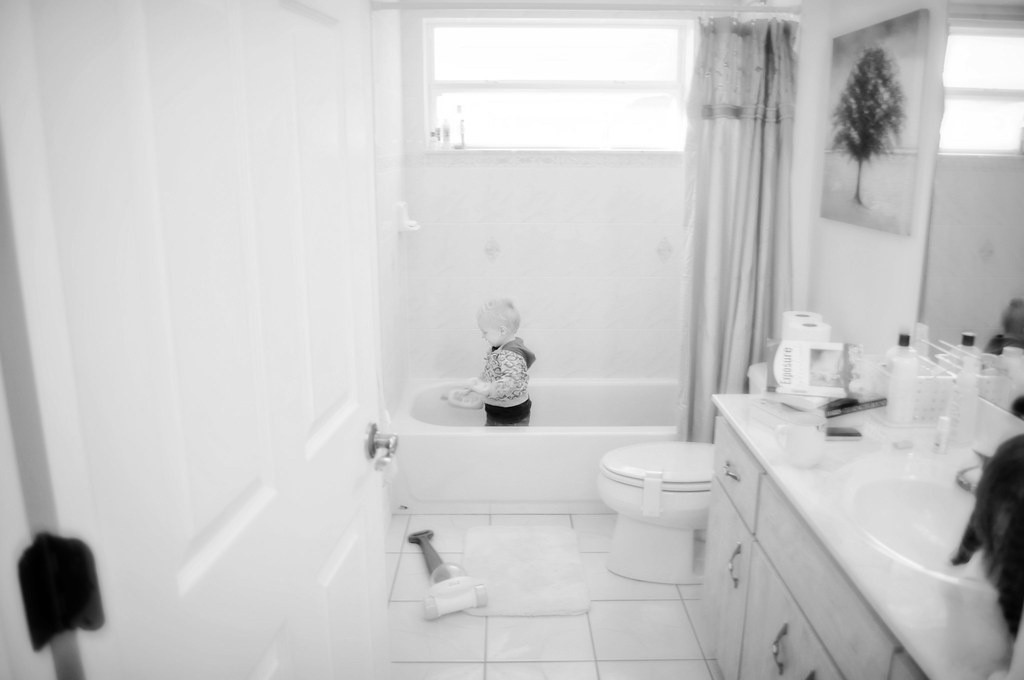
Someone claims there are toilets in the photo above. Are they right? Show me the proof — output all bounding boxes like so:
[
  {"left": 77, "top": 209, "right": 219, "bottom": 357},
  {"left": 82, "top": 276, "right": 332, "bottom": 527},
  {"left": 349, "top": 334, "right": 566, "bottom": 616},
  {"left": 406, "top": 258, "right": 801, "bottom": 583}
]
[{"left": 595, "top": 362, "right": 772, "bottom": 587}]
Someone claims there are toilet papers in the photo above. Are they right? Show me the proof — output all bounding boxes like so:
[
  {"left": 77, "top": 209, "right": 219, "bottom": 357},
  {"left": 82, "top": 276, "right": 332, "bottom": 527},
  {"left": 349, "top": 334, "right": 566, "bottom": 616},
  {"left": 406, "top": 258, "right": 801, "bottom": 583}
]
[
  {"left": 789, "top": 319, "right": 834, "bottom": 344},
  {"left": 781, "top": 311, "right": 826, "bottom": 342}
]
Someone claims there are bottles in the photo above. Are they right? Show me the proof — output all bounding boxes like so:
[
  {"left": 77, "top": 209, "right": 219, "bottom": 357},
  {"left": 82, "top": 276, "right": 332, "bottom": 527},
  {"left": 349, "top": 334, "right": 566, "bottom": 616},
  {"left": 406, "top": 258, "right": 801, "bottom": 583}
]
[
  {"left": 881, "top": 332, "right": 920, "bottom": 423},
  {"left": 946, "top": 331, "right": 982, "bottom": 412}
]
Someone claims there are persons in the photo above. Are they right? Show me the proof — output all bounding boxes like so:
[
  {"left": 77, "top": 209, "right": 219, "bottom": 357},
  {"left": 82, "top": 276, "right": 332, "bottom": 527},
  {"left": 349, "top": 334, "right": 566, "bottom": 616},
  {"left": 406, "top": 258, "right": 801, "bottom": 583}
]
[
  {"left": 463, "top": 296, "right": 536, "bottom": 428},
  {"left": 983, "top": 294, "right": 1024, "bottom": 356}
]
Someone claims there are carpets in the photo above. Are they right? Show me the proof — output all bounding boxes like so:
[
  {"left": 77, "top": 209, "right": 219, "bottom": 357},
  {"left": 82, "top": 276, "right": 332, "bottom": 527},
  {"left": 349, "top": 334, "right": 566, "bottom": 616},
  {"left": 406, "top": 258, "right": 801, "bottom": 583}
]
[{"left": 456, "top": 525, "right": 591, "bottom": 618}]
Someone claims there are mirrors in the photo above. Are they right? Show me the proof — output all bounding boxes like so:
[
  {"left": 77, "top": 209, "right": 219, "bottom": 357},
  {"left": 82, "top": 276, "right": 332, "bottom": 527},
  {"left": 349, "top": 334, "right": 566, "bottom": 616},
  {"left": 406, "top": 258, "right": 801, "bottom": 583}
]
[{"left": 910, "top": 1, "right": 1023, "bottom": 424}]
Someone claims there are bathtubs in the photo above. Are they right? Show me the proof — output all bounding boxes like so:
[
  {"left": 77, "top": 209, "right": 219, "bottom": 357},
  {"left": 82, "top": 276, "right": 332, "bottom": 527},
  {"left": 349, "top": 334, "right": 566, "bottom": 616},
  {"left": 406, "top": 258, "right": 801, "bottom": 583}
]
[{"left": 381, "top": 371, "right": 776, "bottom": 514}]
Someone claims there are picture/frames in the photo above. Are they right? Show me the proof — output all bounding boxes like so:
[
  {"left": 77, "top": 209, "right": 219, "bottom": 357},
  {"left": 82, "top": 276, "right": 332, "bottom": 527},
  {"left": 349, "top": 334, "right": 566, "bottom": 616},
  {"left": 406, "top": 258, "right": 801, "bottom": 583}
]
[{"left": 820, "top": 8, "right": 929, "bottom": 237}]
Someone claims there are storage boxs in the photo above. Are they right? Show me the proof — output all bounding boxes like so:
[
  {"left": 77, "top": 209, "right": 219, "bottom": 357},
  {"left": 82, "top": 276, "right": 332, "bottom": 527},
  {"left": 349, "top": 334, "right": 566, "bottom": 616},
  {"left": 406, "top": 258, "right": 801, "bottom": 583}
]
[
  {"left": 852, "top": 350, "right": 959, "bottom": 429},
  {"left": 932, "top": 352, "right": 1013, "bottom": 412}
]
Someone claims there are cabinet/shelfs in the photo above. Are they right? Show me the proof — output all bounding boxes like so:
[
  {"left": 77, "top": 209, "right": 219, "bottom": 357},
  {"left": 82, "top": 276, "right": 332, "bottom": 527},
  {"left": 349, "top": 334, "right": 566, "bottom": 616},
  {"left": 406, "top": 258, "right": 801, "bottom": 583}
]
[
  {"left": 737, "top": 474, "right": 903, "bottom": 680},
  {"left": 889, "top": 648, "right": 929, "bottom": 679},
  {"left": 700, "top": 414, "right": 766, "bottom": 679}
]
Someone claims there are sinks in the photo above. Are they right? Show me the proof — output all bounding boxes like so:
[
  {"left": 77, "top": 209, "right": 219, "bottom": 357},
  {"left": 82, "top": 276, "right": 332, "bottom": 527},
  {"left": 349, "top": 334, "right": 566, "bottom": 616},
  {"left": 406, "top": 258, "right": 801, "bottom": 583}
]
[{"left": 843, "top": 437, "right": 1009, "bottom": 598}]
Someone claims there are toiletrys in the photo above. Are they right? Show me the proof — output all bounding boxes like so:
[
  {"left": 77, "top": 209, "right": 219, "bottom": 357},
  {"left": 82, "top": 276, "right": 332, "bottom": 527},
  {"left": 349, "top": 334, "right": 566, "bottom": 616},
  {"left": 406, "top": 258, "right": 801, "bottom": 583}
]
[
  {"left": 932, "top": 414, "right": 952, "bottom": 452},
  {"left": 945, "top": 329, "right": 983, "bottom": 397},
  {"left": 879, "top": 334, "right": 919, "bottom": 423}
]
